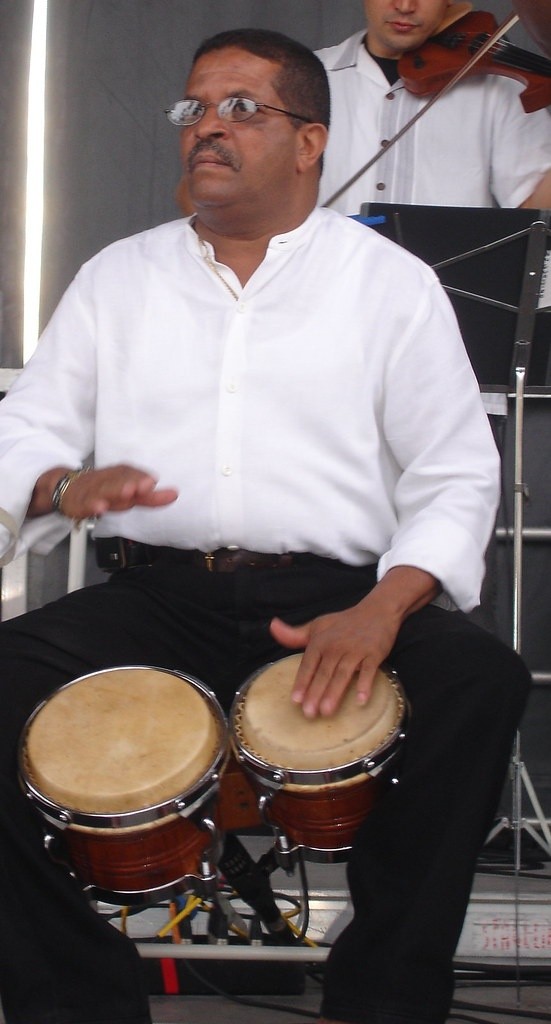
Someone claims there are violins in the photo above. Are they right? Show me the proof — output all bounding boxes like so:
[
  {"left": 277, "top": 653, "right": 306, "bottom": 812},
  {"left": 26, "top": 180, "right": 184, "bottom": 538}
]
[{"left": 397, "top": 10, "right": 551, "bottom": 114}]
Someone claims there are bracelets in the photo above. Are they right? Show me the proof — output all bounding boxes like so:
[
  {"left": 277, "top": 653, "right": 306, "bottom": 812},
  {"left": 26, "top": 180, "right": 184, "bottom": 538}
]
[{"left": 51, "top": 470, "right": 90, "bottom": 510}]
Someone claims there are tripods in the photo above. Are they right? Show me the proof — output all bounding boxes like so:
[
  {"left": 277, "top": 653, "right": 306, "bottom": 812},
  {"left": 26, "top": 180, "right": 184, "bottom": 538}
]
[{"left": 354, "top": 203, "right": 550, "bottom": 1007}]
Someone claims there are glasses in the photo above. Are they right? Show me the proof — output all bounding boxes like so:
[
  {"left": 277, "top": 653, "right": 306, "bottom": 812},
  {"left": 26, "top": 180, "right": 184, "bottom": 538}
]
[{"left": 162, "top": 97, "right": 312, "bottom": 129}]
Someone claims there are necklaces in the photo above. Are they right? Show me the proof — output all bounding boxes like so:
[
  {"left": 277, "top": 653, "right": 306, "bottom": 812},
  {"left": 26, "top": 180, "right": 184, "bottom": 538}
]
[{"left": 192, "top": 226, "right": 239, "bottom": 302}]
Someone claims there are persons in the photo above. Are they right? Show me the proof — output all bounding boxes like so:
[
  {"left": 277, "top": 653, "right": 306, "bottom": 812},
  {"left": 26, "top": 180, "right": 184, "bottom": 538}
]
[
  {"left": 175, "top": 0, "right": 551, "bottom": 873},
  {"left": 0, "top": 27, "right": 533, "bottom": 1024}
]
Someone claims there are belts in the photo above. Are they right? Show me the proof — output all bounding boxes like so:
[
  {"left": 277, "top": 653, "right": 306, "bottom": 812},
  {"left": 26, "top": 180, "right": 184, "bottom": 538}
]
[{"left": 96, "top": 537, "right": 323, "bottom": 573}]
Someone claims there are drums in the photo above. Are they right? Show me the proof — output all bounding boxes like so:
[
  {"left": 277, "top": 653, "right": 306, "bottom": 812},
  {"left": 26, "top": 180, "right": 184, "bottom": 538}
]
[
  {"left": 225, "top": 649, "right": 416, "bottom": 866},
  {"left": 14, "top": 663, "right": 232, "bottom": 909}
]
